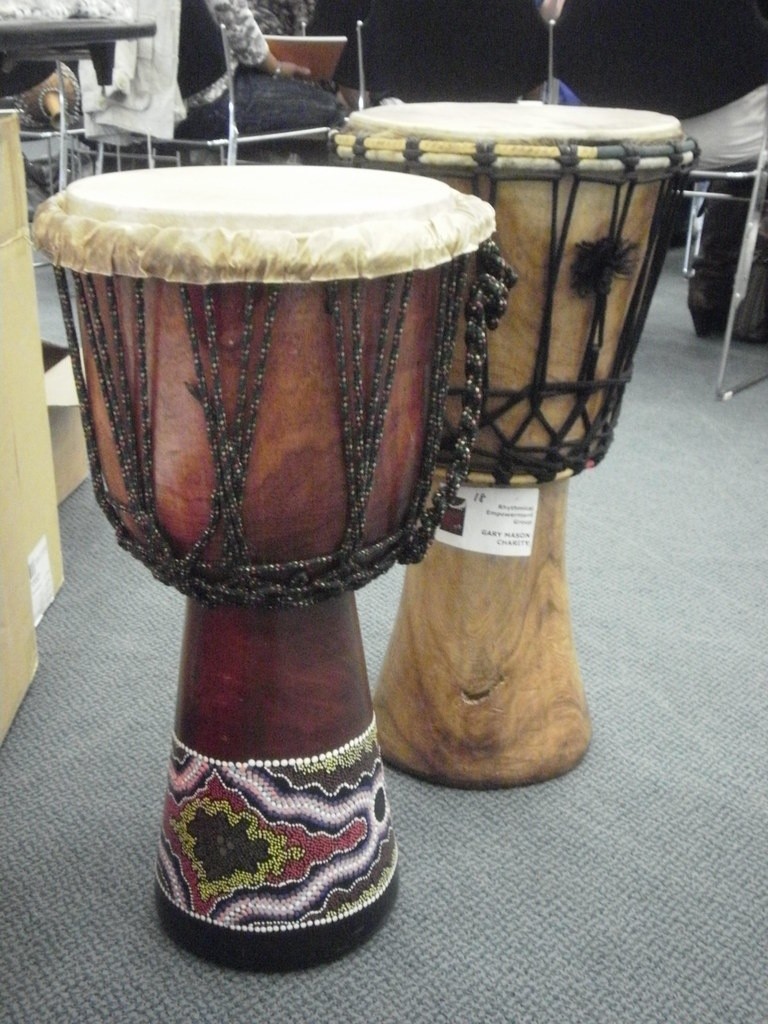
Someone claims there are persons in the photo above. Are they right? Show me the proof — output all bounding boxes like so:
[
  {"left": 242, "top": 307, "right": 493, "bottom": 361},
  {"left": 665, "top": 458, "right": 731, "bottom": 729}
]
[
  {"left": 0, "top": 0, "right": 347, "bottom": 166},
  {"left": 246, "top": 0, "right": 564, "bottom": 110},
  {"left": 563, "top": 1, "right": 767, "bottom": 344}
]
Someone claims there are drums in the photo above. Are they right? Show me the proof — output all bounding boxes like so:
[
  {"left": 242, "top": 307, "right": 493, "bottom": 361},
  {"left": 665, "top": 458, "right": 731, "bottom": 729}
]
[
  {"left": 329, "top": 99, "right": 686, "bottom": 791},
  {"left": 28, "top": 163, "right": 499, "bottom": 978}
]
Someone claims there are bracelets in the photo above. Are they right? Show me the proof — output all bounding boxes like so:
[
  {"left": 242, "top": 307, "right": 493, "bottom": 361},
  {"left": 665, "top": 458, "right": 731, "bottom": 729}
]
[
  {"left": 270, "top": 59, "right": 283, "bottom": 80},
  {"left": 292, "top": 21, "right": 306, "bottom": 29}
]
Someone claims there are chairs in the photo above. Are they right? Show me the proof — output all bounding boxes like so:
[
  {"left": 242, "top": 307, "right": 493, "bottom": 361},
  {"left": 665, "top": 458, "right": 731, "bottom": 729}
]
[
  {"left": 675, "top": 87, "right": 768, "bottom": 399},
  {"left": 51, "top": 1, "right": 331, "bottom": 191}
]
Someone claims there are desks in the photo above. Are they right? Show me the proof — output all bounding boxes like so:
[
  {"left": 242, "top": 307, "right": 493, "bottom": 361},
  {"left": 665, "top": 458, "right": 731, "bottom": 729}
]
[{"left": 0, "top": 17, "right": 156, "bottom": 268}]
